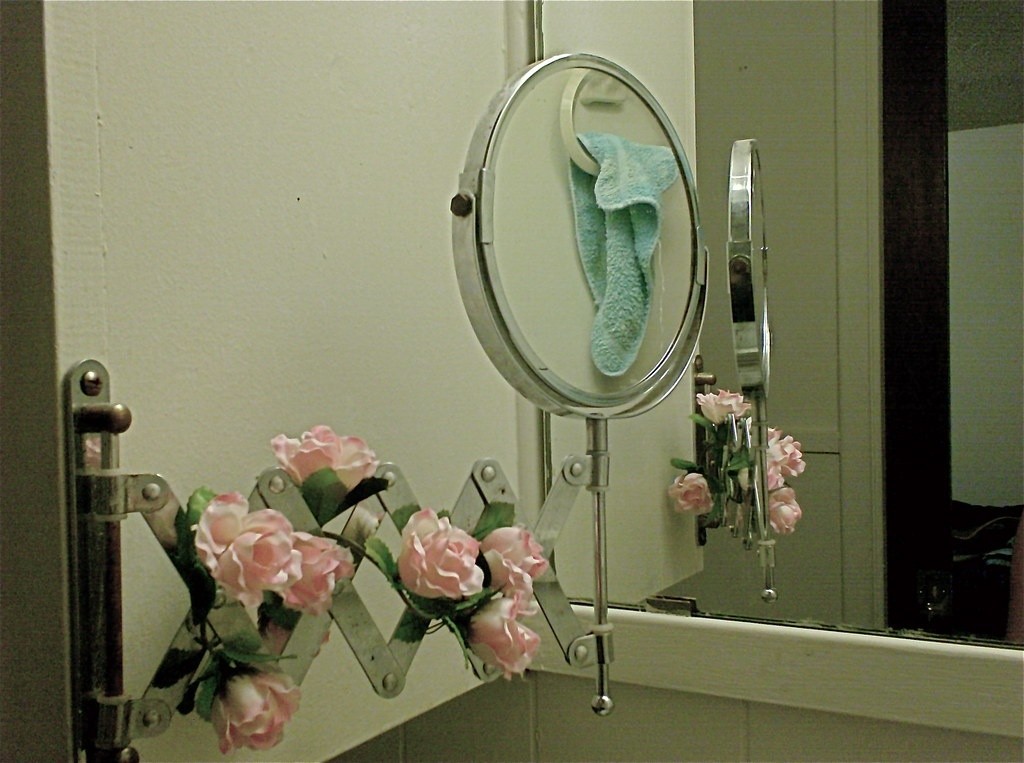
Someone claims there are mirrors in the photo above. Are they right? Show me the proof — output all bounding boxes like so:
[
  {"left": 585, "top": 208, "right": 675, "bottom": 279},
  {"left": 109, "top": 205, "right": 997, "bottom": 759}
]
[
  {"left": 724, "top": 137, "right": 773, "bottom": 401},
  {"left": 447, "top": 52, "right": 706, "bottom": 419},
  {"left": 532, "top": 0, "right": 1024, "bottom": 647}
]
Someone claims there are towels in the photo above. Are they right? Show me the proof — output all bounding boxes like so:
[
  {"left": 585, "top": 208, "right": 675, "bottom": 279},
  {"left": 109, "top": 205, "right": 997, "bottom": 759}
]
[{"left": 568, "top": 132, "right": 679, "bottom": 379}]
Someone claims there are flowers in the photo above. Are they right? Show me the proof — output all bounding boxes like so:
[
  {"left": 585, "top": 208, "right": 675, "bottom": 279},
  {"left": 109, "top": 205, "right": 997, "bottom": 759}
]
[
  {"left": 664, "top": 391, "right": 802, "bottom": 536},
  {"left": 174, "top": 424, "right": 548, "bottom": 756}
]
